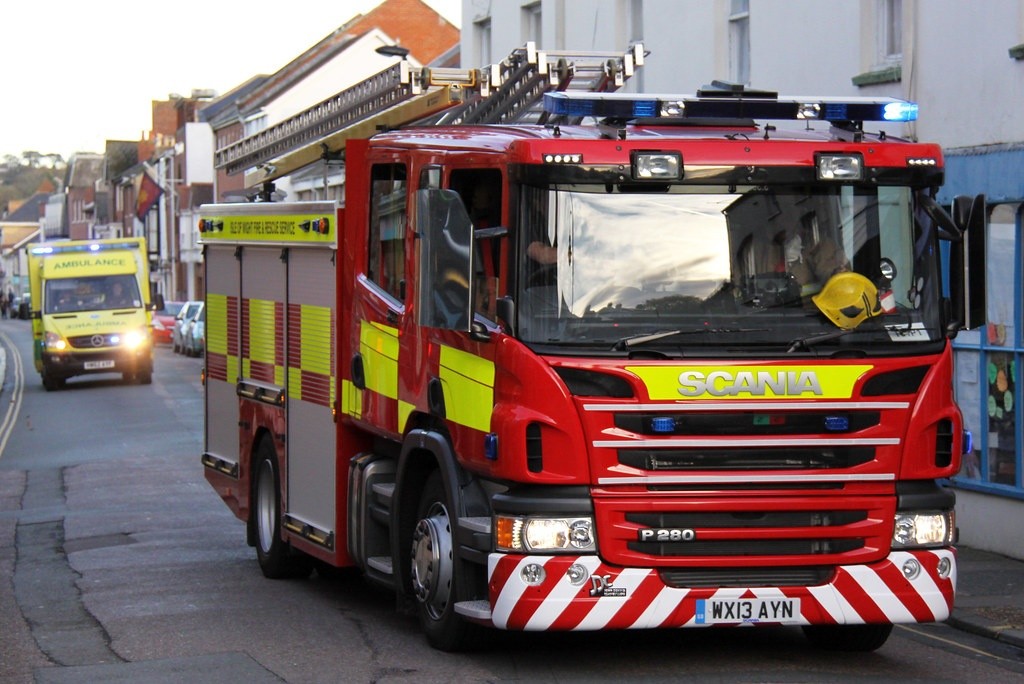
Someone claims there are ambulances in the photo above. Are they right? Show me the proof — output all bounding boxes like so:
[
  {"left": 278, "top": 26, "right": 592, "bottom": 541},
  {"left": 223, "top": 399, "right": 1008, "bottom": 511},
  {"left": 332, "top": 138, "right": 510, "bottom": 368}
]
[{"left": 25, "top": 235, "right": 165, "bottom": 392}]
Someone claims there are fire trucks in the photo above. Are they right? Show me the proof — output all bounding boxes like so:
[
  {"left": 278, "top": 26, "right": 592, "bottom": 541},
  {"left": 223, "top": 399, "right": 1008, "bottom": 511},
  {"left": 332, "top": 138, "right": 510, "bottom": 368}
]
[{"left": 202, "top": 40, "right": 971, "bottom": 652}]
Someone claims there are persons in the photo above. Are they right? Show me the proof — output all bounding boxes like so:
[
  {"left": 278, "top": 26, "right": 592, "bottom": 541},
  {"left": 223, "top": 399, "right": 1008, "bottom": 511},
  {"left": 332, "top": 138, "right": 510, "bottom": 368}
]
[
  {"left": 52, "top": 288, "right": 81, "bottom": 312},
  {"left": 528, "top": 199, "right": 629, "bottom": 294},
  {"left": 0, "top": 288, "right": 14, "bottom": 319},
  {"left": 105, "top": 282, "right": 136, "bottom": 308},
  {"left": 747, "top": 194, "right": 852, "bottom": 305}
]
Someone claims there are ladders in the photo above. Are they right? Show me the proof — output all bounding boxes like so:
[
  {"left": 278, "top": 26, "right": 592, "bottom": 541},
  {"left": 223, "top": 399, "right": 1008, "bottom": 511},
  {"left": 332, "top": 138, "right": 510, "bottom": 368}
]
[
  {"left": 212, "top": 61, "right": 505, "bottom": 177},
  {"left": 425, "top": 41, "right": 651, "bottom": 125}
]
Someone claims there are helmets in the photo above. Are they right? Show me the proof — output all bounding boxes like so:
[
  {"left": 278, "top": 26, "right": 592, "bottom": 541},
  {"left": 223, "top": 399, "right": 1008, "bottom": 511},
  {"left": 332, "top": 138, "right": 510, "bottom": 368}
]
[{"left": 811, "top": 272, "right": 883, "bottom": 330}]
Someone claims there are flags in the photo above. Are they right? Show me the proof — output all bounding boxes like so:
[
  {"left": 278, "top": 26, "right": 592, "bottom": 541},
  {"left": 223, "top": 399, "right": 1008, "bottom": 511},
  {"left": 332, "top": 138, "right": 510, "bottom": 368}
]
[{"left": 135, "top": 170, "right": 163, "bottom": 222}]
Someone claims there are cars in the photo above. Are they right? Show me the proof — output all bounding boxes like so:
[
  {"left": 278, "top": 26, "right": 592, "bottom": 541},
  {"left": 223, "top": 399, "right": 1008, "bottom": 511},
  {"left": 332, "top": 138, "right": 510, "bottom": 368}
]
[
  {"left": 10, "top": 293, "right": 31, "bottom": 320},
  {"left": 152, "top": 301, "right": 205, "bottom": 358}
]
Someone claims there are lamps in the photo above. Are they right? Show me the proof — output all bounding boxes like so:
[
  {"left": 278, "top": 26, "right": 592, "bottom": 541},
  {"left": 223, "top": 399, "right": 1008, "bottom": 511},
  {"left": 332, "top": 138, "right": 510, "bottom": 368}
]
[{"left": 375, "top": 45, "right": 408, "bottom": 62}]
